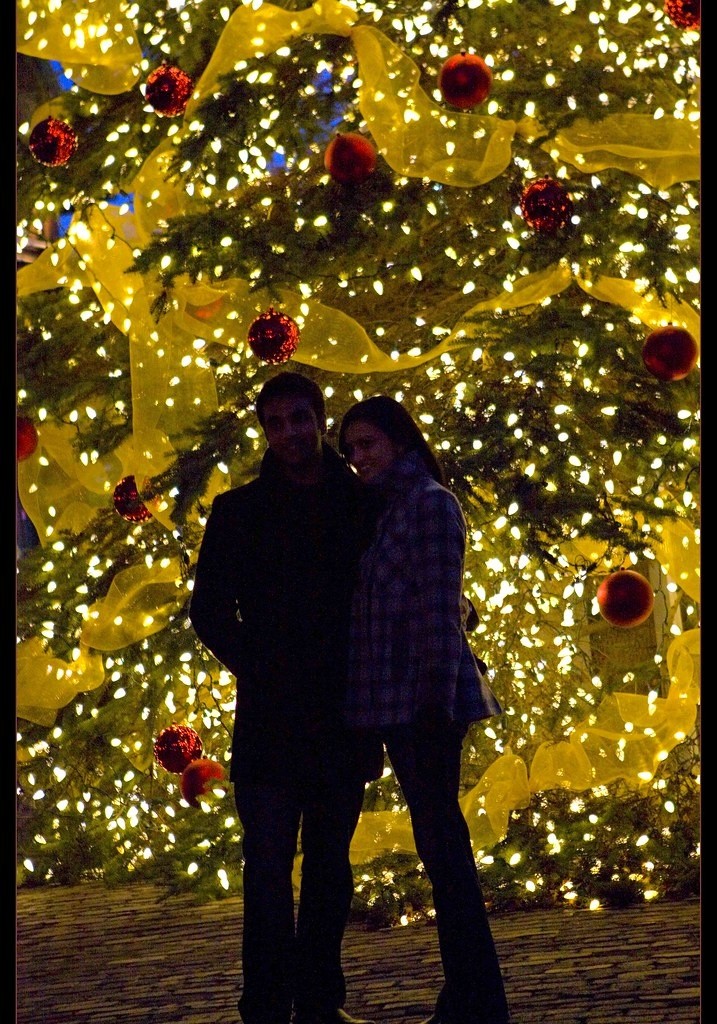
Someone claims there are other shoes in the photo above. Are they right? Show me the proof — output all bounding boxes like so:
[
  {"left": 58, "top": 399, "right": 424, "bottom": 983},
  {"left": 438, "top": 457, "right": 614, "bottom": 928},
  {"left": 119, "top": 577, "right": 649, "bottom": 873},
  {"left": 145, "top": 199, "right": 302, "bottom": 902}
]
[
  {"left": 419, "top": 1012, "right": 447, "bottom": 1024},
  {"left": 291, "top": 1006, "right": 375, "bottom": 1024}
]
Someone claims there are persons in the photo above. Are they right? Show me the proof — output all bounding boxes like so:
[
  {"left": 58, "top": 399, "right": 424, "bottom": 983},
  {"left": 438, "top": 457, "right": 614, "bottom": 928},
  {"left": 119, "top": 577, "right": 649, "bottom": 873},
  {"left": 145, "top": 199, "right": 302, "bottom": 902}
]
[
  {"left": 190, "top": 375, "right": 480, "bottom": 1024},
  {"left": 340, "top": 395, "right": 511, "bottom": 1023}
]
